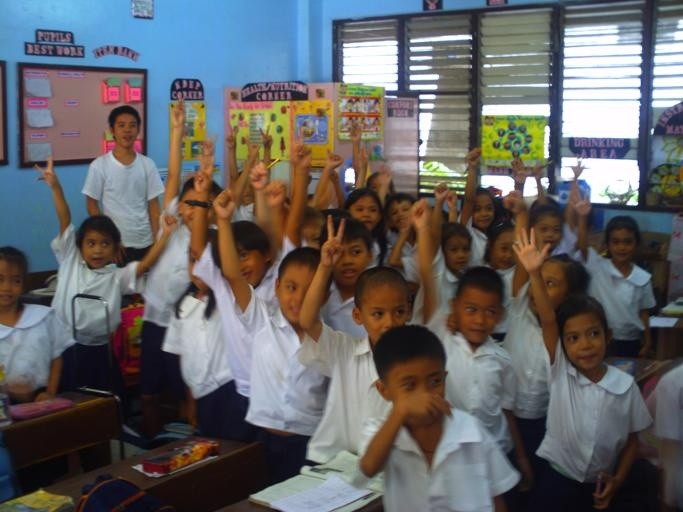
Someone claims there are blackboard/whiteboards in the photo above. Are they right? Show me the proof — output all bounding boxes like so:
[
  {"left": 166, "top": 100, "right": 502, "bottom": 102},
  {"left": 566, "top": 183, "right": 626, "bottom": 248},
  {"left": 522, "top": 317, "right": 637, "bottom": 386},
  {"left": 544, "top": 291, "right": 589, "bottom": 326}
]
[{"left": 17, "top": 62, "right": 148, "bottom": 168}]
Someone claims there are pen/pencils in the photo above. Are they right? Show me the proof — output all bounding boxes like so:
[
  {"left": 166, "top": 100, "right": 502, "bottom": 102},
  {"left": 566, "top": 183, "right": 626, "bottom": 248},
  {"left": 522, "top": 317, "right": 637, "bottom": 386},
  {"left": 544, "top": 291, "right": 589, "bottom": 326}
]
[{"left": 266, "top": 158, "right": 279, "bottom": 169}]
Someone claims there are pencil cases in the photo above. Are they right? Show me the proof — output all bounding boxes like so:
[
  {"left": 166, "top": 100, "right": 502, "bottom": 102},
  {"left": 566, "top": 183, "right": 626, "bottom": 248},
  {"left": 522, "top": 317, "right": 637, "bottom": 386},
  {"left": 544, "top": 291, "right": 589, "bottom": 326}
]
[{"left": 142, "top": 436, "right": 219, "bottom": 474}]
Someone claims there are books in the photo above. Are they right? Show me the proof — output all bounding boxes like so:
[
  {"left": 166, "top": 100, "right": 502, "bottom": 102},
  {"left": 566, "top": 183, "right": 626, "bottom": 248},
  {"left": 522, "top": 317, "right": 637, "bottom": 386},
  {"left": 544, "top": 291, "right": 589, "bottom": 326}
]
[
  {"left": 3, "top": 489, "right": 74, "bottom": 512},
  {"left": 661, "top": 294, "right": 682, "bottom": 319},
  {"left": 648, "top": 313, "right": 679, "bottom": 329}
]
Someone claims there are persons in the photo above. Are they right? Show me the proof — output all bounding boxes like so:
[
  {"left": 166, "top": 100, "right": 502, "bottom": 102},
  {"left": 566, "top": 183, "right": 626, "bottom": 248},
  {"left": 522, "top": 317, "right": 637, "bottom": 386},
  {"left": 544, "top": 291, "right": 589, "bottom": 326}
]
[
  {"left": 30, "top": 150, "right": 177, "bottom": 444},
  {"left": 0, "top": 242, "right": 77, "bottom": 506},
  {"left": 77, "top": 105, "right": 167, "bottom": 312},
  {"left": 654, "top": 361, "right": 682, "bottom": 512},
  {"left": 136, "top": 94, "right": 659, "bottom": 510}
]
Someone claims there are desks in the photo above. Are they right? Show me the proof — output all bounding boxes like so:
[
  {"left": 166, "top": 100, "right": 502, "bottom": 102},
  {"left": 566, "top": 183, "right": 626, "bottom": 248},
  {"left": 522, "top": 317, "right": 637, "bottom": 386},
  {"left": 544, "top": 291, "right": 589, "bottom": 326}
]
[
  {"left": 1, "top": 380, "right": 383, "bottom": 512},
  {"left": 603, "top": 295, "right": 683, "bottom": 386}
]
